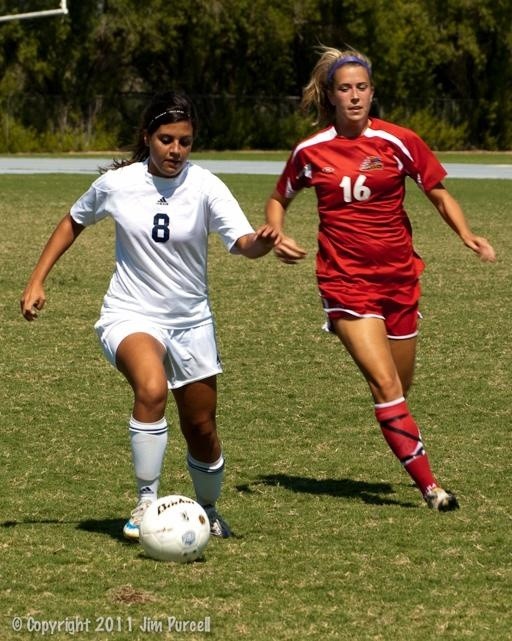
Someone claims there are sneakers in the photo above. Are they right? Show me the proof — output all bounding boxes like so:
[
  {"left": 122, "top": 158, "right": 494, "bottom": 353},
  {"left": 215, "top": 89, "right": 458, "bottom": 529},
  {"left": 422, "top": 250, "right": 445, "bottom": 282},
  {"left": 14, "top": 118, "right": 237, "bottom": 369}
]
[
  {"left": 122, "top": 500, "right": 153, "bottom": 538},
  {"left": 207, "top": 511, "right": 233, "bottom": 540},
  {"left": 425, "top": 488, "right": 459, "bottom": 511}
]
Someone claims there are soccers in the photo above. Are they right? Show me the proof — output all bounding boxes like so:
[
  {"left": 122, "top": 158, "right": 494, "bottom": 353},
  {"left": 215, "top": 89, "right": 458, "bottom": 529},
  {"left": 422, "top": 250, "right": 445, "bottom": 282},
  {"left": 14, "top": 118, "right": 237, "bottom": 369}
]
[{"left": 138, "top": 495, "right": 212, "bottom": 564}]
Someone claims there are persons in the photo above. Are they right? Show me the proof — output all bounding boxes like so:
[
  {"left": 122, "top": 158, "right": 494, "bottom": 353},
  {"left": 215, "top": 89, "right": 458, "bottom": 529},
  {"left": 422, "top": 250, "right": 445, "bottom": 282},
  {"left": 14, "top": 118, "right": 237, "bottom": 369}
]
[
  {"left": 265, "top": 38, "right": 499, "bottom": 514},
  {"left": 19, "top": 87, "right": 280, "bottom": 545}
]
